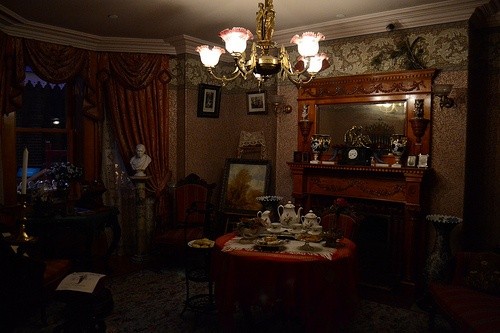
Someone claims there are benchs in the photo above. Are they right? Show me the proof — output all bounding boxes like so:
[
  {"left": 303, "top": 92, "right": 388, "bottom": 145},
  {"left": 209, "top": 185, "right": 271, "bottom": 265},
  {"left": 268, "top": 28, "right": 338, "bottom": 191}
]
[{"left": 423, "top": 250, "right": 500, "bottom": 333}]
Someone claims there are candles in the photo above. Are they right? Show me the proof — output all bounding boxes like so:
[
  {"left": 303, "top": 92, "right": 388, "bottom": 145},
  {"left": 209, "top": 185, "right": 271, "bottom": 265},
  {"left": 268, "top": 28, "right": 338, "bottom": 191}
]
[{"left": 22, "top": 147, "right": 28, "bottom": 194}]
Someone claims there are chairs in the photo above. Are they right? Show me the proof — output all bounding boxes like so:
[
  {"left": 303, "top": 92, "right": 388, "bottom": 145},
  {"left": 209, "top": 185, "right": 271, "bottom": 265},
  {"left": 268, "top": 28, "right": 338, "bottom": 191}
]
[
  {"left": 178, "top": 200, "right": 217, "bottom": 316},
  {"left": 321, "top": 198, "right": 364, "bottom": 239},
  {"left": 154, "top": 173, "right": 217, "bottom": 274}
]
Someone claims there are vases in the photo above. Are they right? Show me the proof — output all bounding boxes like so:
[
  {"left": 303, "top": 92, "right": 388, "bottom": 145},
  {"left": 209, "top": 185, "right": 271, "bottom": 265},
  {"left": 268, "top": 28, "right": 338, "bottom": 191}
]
[
  {"left": 56, "top": 189, "right": 71, "bottom": 217},
  {"left": 310, "top": 134, "right": 322, "bottom": 164},
  {"left": 391, "top": 134, "right": 406, "bottom": 168},
  {"left": 322, "top": 135, "right": 331, "bottom": 154},
  {"left": 426, "top": 214, "right": 464, "bottom": 285}
]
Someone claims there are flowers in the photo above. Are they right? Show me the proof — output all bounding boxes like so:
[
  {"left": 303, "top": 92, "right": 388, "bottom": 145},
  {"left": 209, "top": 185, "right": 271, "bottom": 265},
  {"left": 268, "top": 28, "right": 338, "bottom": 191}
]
[{"left": 44, "top": 160, "right": 83, "bottom": 190}]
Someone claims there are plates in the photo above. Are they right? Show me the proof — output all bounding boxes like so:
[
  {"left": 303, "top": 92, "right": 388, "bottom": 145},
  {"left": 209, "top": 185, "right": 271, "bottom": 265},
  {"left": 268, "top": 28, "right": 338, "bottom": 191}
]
[
  {"left": 258, "top": 229, "right": 324, "bottom": 247},
  {"left": 188, "top": 240, "right": 215, "bottom": 248}
]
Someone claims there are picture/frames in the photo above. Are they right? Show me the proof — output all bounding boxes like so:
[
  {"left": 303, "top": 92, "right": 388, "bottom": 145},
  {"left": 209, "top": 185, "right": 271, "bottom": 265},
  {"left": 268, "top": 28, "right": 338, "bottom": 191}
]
[
  {"left": 246, "top": 90, "right": 268, "bottom": 115},
  {"left": 219, "top": 158, "right": 272, "bottom": 217},
  {"left": 197, "top": 84, "right": 222, "bottom": 118}
]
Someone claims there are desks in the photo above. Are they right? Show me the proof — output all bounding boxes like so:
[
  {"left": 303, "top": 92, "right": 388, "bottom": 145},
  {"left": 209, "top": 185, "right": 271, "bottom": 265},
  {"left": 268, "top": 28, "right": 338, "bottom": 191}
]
[
  {"left": 30, "top": 205, "right": 121, "bottom": 264},
  {"left": 284, "top": 162, "right": 433, "bottom": 308}
]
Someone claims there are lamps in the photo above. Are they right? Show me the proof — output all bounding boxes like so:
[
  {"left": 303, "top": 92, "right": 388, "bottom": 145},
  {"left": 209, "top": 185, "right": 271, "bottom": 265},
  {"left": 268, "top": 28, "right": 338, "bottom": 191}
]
[
  {"left": 267, "top": 94, "right": 292, "bottom": 116},
  {"left": 194, "top": 0, "right": 329, "bottom": 89},
  {"left": 430, "top": 84, "right": 454, "bottom": 109}
]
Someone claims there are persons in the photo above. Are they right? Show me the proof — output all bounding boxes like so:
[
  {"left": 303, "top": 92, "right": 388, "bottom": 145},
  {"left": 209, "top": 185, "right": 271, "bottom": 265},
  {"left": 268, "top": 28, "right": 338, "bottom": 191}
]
[
  {"left": 206, "top": 93, "right": 215, "bottom": 109},
  {"left": 131, "top": 144, "right": 152, "bottom": 171},
  {"left": 51, "top": 159, "right": 80, "bottom": 208}
]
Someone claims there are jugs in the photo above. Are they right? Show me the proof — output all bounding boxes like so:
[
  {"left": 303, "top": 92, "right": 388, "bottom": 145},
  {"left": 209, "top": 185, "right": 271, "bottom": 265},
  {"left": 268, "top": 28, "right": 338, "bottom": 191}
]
[
  {"left": 257, "top": 210, "right": 271, "bottom": 226},
  {"left": 301, "top": 210, "right": 322, "bottom": 228}
]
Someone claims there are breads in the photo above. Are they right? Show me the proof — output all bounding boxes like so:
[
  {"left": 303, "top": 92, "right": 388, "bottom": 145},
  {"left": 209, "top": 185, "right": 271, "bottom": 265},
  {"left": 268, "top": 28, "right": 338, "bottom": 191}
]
[{"left": 191, "top": 238, "right": 215, "bottom": 248}]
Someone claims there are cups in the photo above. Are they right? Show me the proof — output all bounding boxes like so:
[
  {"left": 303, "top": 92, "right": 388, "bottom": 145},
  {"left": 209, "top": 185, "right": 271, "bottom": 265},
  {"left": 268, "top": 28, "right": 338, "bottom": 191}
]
[{"left": 271, "top": 223, "right": 322, "bottom": 233}]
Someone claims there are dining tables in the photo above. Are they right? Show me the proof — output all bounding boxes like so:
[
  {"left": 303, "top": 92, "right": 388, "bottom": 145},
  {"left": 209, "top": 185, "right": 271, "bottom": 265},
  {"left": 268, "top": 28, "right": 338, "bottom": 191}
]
[{"left": 216, "top": 231, "right": 363, "bottom": 333}]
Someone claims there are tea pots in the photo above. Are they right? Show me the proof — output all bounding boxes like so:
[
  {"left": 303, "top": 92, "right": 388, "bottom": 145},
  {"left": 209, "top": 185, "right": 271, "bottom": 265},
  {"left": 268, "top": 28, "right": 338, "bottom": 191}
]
[{"left": 278, "top": 200, "right": 303, "bottom": 227}]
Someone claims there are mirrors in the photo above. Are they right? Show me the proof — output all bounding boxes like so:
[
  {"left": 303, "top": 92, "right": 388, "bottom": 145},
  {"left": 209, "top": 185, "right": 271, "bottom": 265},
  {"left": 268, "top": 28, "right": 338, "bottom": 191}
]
[{"left": 316, "top": 100, "right": 406, "bottom": 146}]
